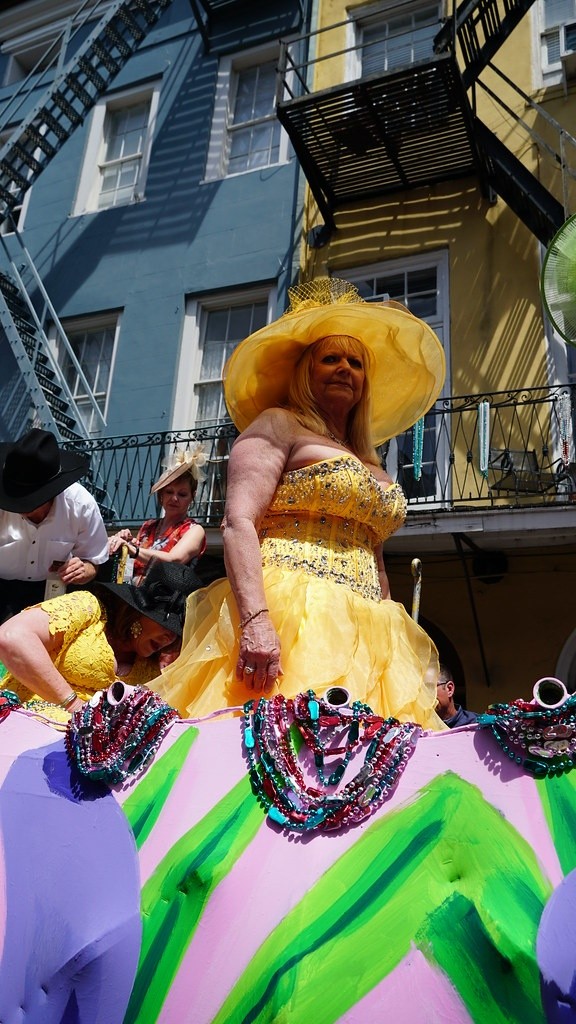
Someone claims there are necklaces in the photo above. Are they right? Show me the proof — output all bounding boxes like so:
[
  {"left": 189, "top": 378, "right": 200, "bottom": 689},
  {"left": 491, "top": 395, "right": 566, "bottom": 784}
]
[
  {"left": 244, "top": 690, "right": 421, "bottom": 835},
  {"left": 485, "top": 696, "right": 576, "bottom": 774},
  {"left": 329, "top": 431, "right": 348, "bottom": 448},
  {"left": 64, "top": 687, "right": 183, "bottom": 784}
]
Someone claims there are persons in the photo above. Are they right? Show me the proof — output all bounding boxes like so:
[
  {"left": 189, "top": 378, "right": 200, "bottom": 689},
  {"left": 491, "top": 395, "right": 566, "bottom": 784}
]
[
  {"left": 424, "top": 662, "right": 478, "bottom": 728},
  {"left": 0, "top": 554, "right": 202, "bottom": 730},
  {"left": 0, "top": 428, "right": 109, "bottom": 585},
  {"left": 107, "top": 444, "right": 210, "bottom": 667},
  {"left": 144, "top": 279, "right": 451, "bottom": 728}
]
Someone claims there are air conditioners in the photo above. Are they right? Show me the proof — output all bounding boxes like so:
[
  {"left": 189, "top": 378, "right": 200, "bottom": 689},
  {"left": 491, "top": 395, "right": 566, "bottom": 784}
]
[{"left": 559, "top": 18, "right": 576, "bottom": 81}]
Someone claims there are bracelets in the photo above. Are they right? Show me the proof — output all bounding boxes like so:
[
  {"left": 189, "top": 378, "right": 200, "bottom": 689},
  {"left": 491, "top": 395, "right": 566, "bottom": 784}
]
[
  {"left": 129, "top": 545, "right": 139, "bottom": 559},
  {"left": 238, "top": 608, "right": 269, "bottom": 628},
  {"left": 58, "top": 693, "right": 77, "bottom": 708}
]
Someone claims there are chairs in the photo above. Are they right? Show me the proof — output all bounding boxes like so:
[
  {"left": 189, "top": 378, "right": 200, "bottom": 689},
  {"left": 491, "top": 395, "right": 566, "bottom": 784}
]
[{"left": 485, "top": 448, "right": 575, "bottom": 506}]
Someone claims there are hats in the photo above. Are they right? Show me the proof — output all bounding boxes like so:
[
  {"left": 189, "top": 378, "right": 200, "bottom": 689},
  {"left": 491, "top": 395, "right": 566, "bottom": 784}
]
[
  {"left": 148, "top": 444, "right": 209, "bottom": 496},
  {"left": 222, "top": 278, "right": 446, "bottom": 448},
  {"left": 93, "top": 562, "right": 205, "bottom": 636},
  {"left": 0, "top": 427, "right": 93, "bottom": 513}
]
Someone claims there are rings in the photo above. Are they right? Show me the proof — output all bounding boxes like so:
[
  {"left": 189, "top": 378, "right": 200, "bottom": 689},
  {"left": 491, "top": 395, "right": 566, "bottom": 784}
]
[{"left": 244, "top": 666, "right": 255, "bottom": 675}]
